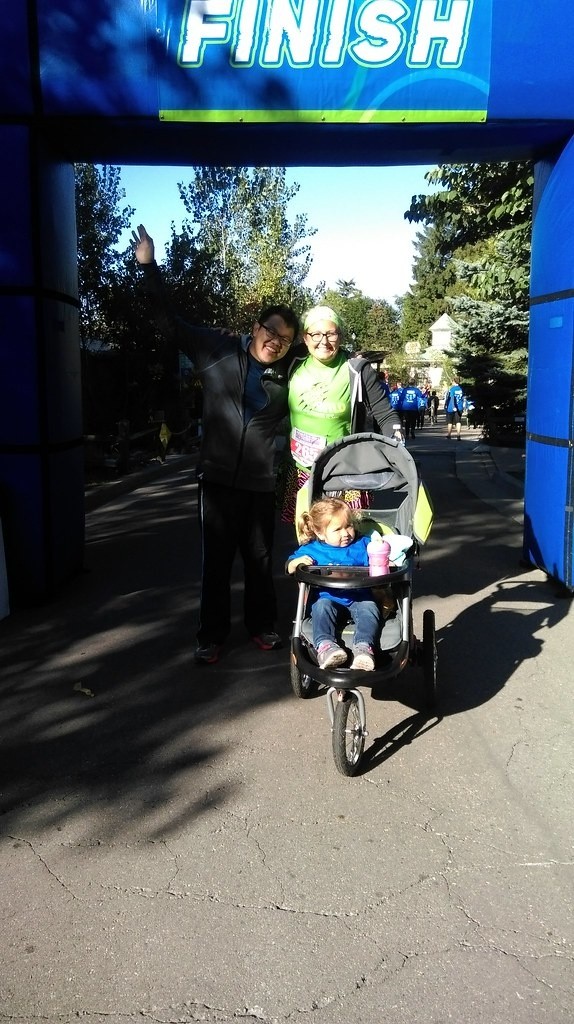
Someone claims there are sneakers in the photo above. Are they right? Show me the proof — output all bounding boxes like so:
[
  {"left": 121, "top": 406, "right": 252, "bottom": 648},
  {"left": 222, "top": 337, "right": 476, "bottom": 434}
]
[
  {"left": 349, "top": 641, "right": 375, "bottom": 671},
  {"left": 245, "top": 622, "right": 284, "bottom": 650},
  {"left": 317, "top": 640, "right": 348, "bottom": 669},
  {"left": 192, "top": 625, "right": 223, "bottom": 666}
]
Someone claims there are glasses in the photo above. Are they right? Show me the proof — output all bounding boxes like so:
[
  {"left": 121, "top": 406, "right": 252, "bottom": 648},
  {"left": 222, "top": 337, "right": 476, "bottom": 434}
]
[
  {"left": 259, "top": 322, "right": 293, "bottom": 347},
  {"left": 307, "top": 332, "right": 341, "bottom": 342}
]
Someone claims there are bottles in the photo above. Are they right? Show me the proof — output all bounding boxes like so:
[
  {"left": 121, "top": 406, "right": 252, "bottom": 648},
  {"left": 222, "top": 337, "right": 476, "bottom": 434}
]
[
  {"left": 366, "top": 530, "right": 390, "bottom": 577},
  {"left": 390, "top": 424, "right": 405, "bottom": 448}
]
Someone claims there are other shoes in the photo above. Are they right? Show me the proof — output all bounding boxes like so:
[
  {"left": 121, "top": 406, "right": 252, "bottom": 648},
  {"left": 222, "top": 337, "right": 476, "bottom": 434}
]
[
  {"left": 447, "top": 435, "right": 451, "bottom": 440},
  {"left": 405, "top": 435, "right": 409, "bottom": 440},
  {"left": 411, "top": 431, "right": 416, "bottom": 439},
  {"left": 457, "top": 437, "right": 461, "bottom": 441}
]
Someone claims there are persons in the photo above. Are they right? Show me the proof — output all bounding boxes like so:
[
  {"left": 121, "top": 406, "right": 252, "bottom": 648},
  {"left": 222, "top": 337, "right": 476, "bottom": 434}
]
[
  {"left": 129, "top": 223, "right": 300, "bottom": 665},
  {"left": 270, "top": 304, "right": 405, "bottom": 643},
  {"left": 373, "top": 371, "right": 476, "bottom": 440},
  {"left": 285, "top": 496, "right": 382, "bottom": 672}
]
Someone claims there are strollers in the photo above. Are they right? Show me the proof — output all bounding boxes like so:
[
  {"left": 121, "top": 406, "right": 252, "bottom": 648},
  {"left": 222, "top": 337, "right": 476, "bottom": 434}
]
[{"left": 286, "top": 432, "right": 436, "bottom": 777}]
